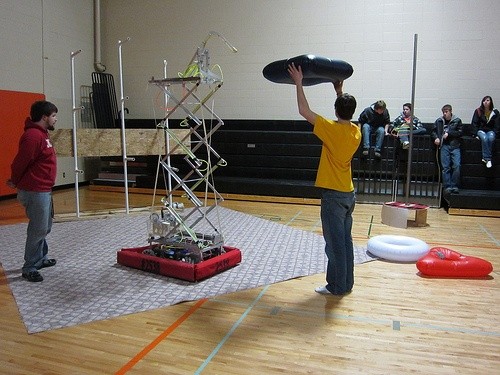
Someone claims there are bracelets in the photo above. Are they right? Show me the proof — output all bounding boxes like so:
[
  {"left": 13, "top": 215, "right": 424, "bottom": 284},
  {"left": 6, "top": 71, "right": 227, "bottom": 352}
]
[{"left": 336, "top": 91, "right": 343, "bottom": 95}]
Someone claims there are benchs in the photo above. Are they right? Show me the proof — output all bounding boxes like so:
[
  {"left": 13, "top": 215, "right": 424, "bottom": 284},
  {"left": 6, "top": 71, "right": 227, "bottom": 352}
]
[{"left": 91, "top": 118, "right": 500, "bottom": 210}]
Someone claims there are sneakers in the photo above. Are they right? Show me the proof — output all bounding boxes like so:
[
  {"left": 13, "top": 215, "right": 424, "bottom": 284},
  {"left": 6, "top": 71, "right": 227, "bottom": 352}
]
[
  {"left": 374, "top": 151, "right": 381, "bottom": 157},
  {"left": 363, "top": 148, "right": 369, "bottom": 155}
]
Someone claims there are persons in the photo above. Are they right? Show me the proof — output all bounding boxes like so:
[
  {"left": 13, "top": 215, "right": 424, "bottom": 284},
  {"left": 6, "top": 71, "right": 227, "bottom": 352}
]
[
  {"left": 470, "top": 96, "right": 500, "bottom": 168},
  {"left": 390, "top": 103, "right": 426, "bottom": 149},
  {"left": 6, "top": 100, "right": 59, "bottom": 282},
  {"left": 358, "top": 100, "right": 391, "bottom": 156},
  {"left": 430, "top": 104, "right": 463, "bottom": 194},
  {"left": 288, "top": 62, "right": 363, "bottom": 296}
]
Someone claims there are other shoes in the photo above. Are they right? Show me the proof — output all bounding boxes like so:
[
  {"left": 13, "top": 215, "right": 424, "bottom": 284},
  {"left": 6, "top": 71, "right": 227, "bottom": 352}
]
[
  {"left": 37, "top": 259, "right": 56, "bottom": 270},
  {"left": 482, "top": 159, "right": 492, "bottom": 168},
  {"left": 445, "top": 187, "right": 459, "bottom": 195},
  {"left": 403, "top": 141, "right": 409, "bottom": 149},
  {"left": 22, "top": 270, "right": 44, "bottom": 282}
]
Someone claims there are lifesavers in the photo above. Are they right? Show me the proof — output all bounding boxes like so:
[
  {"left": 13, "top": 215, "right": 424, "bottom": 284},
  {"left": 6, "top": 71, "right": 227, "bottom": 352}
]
[
  {"left": 367, "top": 234, "right": 429, "bottom": 263},
  {"left": 263, "top": 53, "right": 353, "bottom": 90},
  {"left": 417, "top": 246, "right": 493, "bottom": 276}
]
